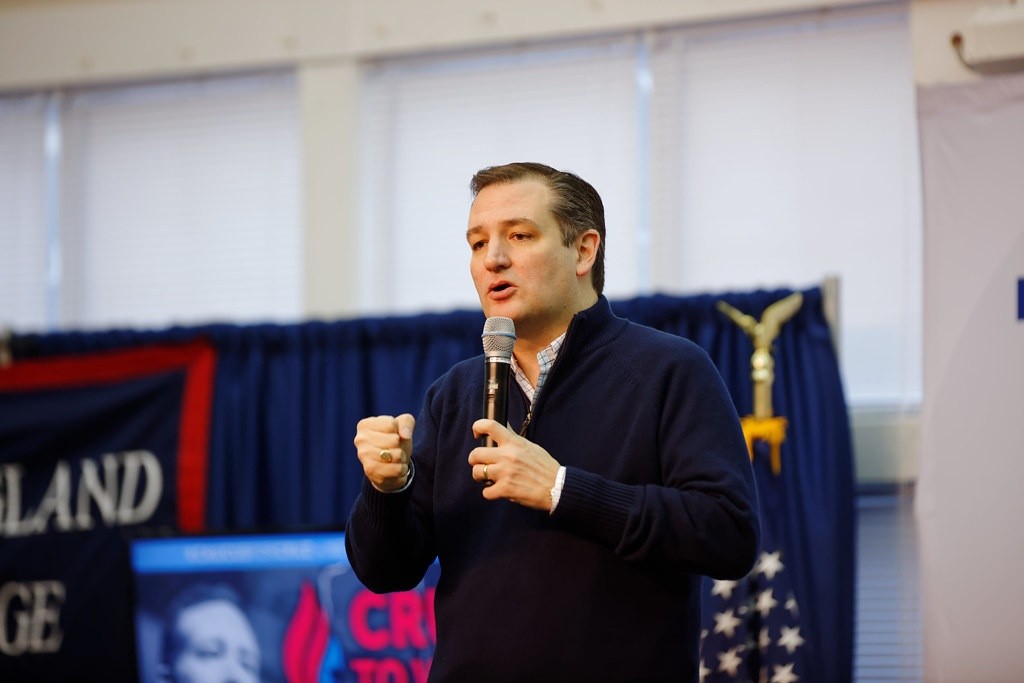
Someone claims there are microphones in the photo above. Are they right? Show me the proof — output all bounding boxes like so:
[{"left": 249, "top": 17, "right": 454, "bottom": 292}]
[{"left": 481, "top": 315, "right": 516, "bottom": 488}]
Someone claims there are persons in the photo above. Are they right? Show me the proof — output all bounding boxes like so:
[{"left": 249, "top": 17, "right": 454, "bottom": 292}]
[
  {"left": 154, "top": 576, "right": 265, "bottom": 683},
  {"left": 343, "top": 161, "right": 761, "bottom": 683}
]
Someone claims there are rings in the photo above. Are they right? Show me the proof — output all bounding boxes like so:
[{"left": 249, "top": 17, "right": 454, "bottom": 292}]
[
  {"left": 483, "top": 463, "right": 490, "bottom": 479},
  {"left": 380, "top": 448, "right": 392, "bottom": 464}
]
